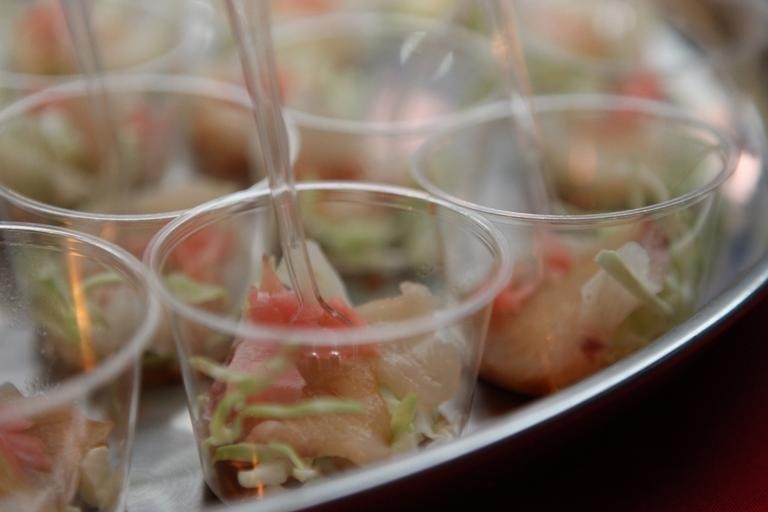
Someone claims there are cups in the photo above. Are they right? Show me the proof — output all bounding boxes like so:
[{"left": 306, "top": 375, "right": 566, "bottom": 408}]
[{"left": 0, "top": 3, "right": 739, "bottom": 510}]
